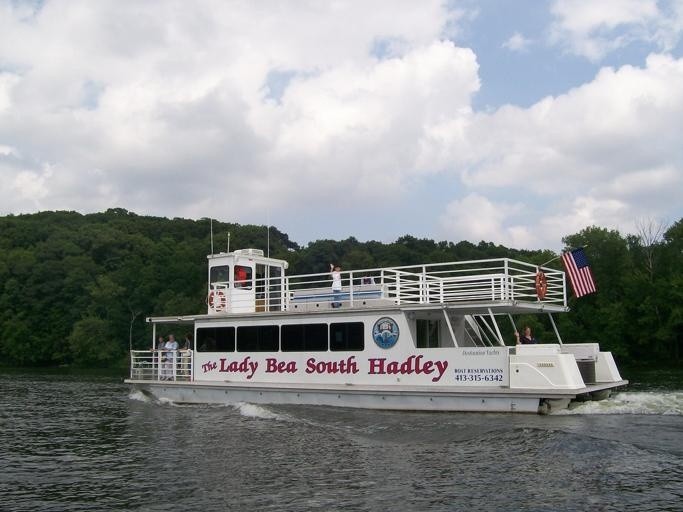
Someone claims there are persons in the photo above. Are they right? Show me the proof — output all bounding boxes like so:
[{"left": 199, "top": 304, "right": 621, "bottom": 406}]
[
  {"left": 329, "top": 263, "right": 341, "bottom": 307},
  {"left": 149, "top": 336, "right": 165, "bottom": 380},
  {"left": 164, "top": 334, "right": 179, "bottom": 381},
  {"left": 514, "top": 327, "right": 538, "bottom": 344},
  {"left": 178, "top": 333, "right": 192, "bottom": 381}
]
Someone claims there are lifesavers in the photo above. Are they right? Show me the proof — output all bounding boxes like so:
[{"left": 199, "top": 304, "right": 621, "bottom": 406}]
[
  {"left": 208, "top": 291, "right": 227, "bottom": 312},
  {"left": 535, "top": 271, "right": 547, "bottom": 299}
]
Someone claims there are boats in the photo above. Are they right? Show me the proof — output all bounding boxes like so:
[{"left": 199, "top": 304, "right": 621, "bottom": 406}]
[{"left": 121, "top": 220, "right": 630, "bottom": 415}]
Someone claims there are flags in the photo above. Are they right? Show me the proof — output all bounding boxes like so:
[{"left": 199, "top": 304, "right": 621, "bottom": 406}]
[{"left": 560, "top": 247, "right": 597, "bottom": 299}]
